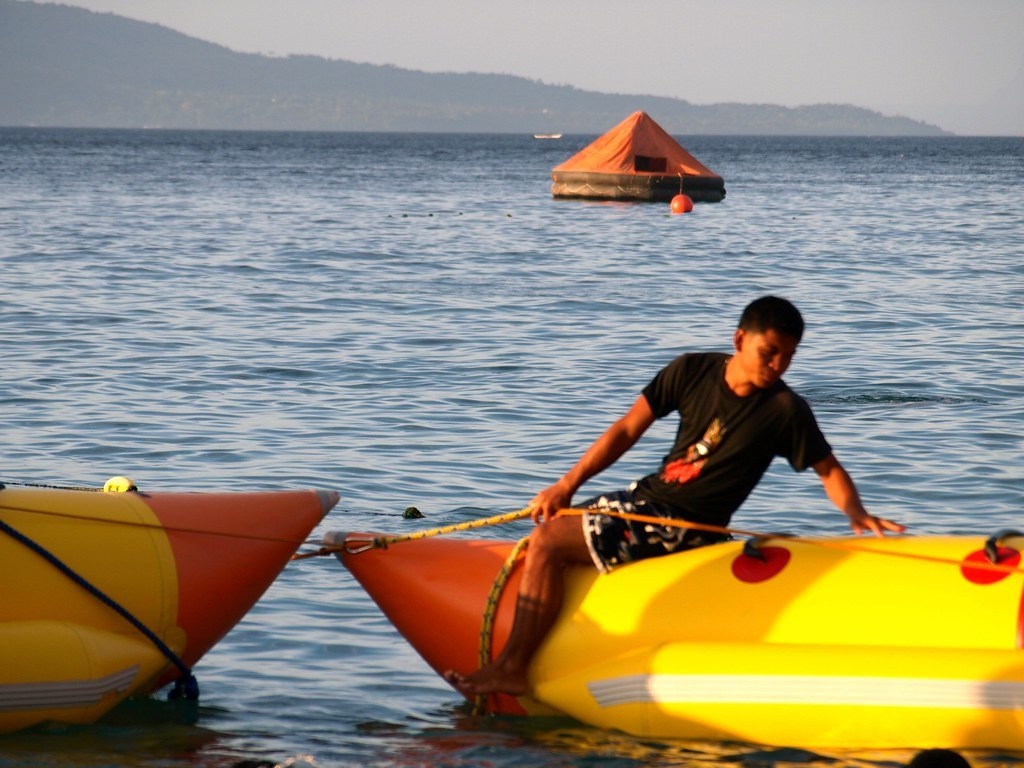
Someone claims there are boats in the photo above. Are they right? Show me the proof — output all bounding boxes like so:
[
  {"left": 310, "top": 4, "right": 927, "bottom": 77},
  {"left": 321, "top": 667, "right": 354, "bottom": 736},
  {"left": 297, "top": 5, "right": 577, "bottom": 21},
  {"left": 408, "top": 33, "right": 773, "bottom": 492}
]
[
  {"left": 316, "top": 516, "right": 1022, "bottom": 759},
  {"left": 545, "top": 110, "right": 728, "bottom": 203},
  {"left": 0, "top": 478, "right": 340, "bottom": 737}
]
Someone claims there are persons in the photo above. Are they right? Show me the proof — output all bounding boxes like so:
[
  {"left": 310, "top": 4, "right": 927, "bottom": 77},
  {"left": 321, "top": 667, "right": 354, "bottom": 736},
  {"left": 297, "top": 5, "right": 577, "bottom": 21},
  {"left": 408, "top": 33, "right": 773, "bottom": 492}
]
[{"left": 440, "top": 296, "right": 907, "bottom": 696}]
